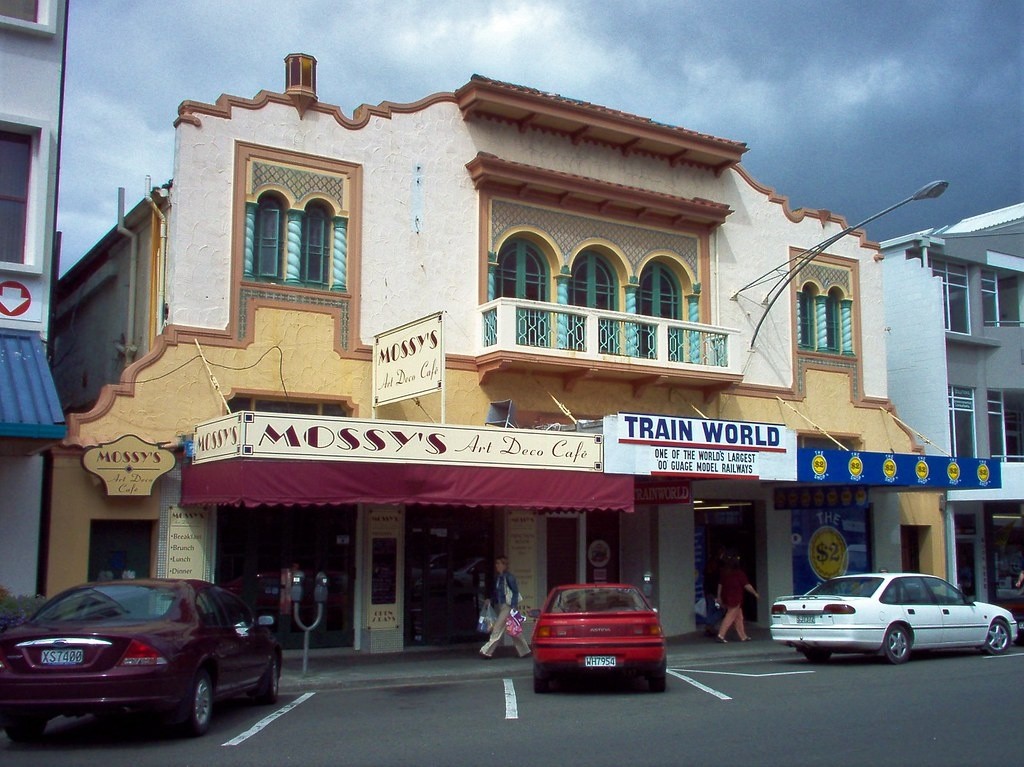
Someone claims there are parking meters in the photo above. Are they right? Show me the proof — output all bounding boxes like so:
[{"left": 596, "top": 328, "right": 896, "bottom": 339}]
[
  {"left": 289, "top": 572, "right": 330, "bottom": 679},
  {"left": 642, "top": 571, "right": 653, "bottom": 600}
]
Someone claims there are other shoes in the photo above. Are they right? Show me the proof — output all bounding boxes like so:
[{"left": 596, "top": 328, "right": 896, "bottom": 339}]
[
  {"left": 517, "top": 652, "right": 531, "bottom": 658},
  {"left": 479, "top": 652, "right": 492, "bottom": 660}
]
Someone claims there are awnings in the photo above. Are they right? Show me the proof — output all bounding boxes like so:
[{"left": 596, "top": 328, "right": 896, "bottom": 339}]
[
  {"left": 182, "top": 411, "right": 1002, "bottom": 517},
  {"left": 0, "top": 327, "right": 67, "bottom": 457}
]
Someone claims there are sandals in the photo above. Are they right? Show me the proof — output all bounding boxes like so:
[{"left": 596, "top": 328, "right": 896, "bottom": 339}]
[
  {"left": 716, "top": 635, "right": 727, "bottom": 643},
  {"left": 741, "top": 636, "right": 753, "bottom": 642}
]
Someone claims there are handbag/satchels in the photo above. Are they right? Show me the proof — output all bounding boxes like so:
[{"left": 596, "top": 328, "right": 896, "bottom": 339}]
[
  {"left": 504, "top": 583, "right": 524, "bottom": 606},
  {"left": 475, "top": 599, "right": 498, "bottom": 634}
]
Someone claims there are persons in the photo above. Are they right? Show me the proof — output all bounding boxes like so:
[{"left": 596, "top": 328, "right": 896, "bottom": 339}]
[
  {"left": 715, "top": 551, "right": 759, "bottom": 642},
  {"left": 473, "top": 557, "right": 531, "bottom": 660},
  {"left": 702, "top": 546, "right": 726, "bottom": 637}
]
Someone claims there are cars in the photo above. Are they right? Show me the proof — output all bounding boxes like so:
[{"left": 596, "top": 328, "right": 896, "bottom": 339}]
[
  {"left": 769, "top": 572, "right": 1019, "bottom": 665},
  {"left": 0, "top": 575, "right": 284, "bottom": 746},
  {"left": 525, "top": 582, "right": 668, "bottom": 694}
]
